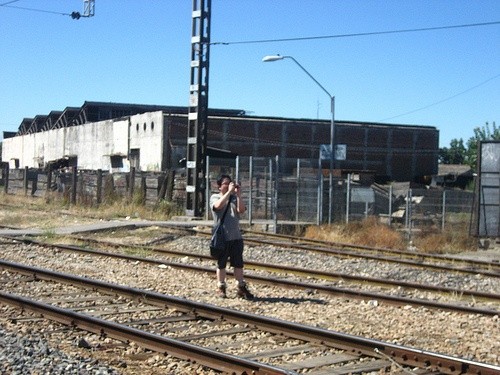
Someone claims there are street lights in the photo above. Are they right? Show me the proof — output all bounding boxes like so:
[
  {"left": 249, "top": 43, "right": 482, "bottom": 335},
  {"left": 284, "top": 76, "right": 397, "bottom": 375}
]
[{"left": 263, "top": 53, "right": 337, "bottom": 227}]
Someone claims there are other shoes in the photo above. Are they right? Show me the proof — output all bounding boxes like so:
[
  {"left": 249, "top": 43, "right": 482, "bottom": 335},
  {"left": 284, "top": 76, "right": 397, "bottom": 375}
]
[
  {"left": 237, "top": 283, "right": 253, "bottom": 300},
  {"left": 218, "top": 286, "right": 226, "bottom": 297}
]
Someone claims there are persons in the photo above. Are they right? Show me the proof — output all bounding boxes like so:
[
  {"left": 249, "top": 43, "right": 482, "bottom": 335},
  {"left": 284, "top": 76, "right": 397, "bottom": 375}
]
[{"left": 209, "top": 175, "right": 254, "bottom": 301}]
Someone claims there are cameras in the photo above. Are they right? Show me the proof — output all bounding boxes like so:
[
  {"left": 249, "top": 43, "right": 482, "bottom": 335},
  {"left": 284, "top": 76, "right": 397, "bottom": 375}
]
[{"left": 233, "top": 184, "right": 239, "bottom": 188}]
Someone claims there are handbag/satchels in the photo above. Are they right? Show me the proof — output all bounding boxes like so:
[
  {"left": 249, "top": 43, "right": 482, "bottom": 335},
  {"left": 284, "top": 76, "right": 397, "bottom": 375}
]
[{"left": 209, "top": 224, "right": 226, "bottom": 258}]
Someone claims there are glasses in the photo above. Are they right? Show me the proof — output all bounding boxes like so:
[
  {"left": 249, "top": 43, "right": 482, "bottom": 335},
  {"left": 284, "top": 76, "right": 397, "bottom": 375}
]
[{"left": 220, "top": 182, "right": 229, "bottom": 185}]
